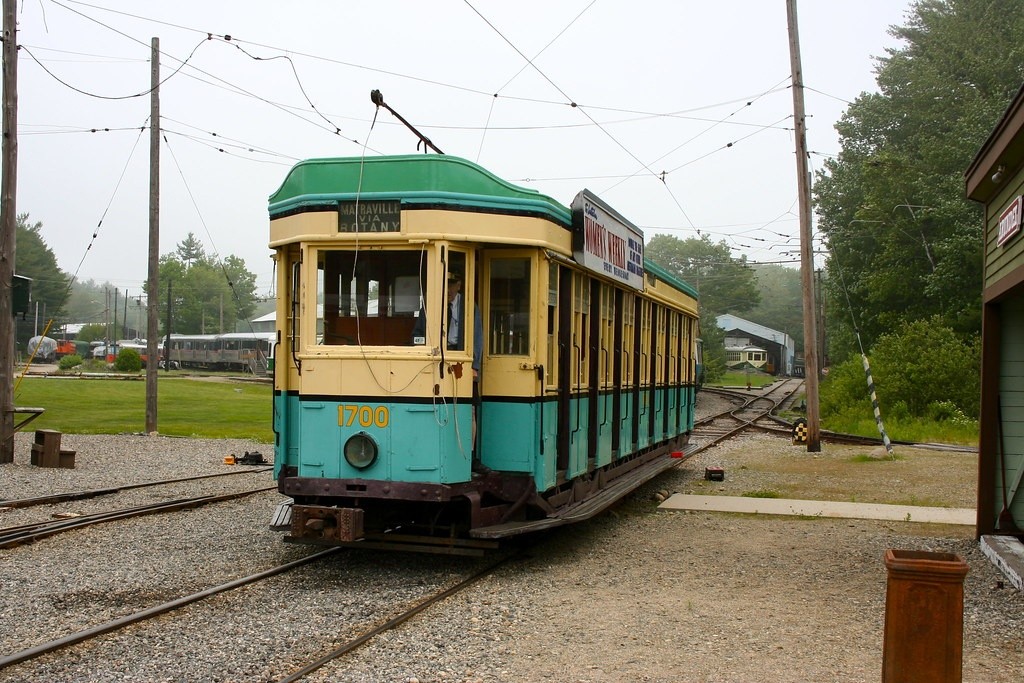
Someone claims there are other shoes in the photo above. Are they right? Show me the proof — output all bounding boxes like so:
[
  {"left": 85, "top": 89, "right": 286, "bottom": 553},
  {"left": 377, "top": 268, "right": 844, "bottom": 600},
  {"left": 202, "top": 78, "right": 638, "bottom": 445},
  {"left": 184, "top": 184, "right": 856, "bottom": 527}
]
[{"left": 472, "top": 458, "right": 491, "bottom": 474}]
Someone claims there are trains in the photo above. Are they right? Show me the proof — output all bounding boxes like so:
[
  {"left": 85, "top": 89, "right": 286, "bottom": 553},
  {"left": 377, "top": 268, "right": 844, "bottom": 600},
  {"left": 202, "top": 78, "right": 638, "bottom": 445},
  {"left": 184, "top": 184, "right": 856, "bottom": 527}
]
[
  {"left": 724, "top": 343, "right": 767, "bottom": 374},
  {"left": 161, "top": 333, "right": 275, "bottom": 379},
  {"left": 266, "top": 153, "right": 699, "bottom": 554}
]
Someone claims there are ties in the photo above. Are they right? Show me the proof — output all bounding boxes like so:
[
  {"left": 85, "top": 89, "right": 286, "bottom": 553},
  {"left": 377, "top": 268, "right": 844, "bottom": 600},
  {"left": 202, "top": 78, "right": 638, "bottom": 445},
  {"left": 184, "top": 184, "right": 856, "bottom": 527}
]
[{"left": 447, "top": 303, "right": 452, "bottom": 342}]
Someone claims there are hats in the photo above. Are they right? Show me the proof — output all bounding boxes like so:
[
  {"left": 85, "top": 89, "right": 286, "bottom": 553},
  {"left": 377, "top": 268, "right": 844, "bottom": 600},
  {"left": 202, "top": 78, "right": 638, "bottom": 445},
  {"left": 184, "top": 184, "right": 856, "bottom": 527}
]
[{"left": 447, "top": 269, "right": 463, "bottom": 279}]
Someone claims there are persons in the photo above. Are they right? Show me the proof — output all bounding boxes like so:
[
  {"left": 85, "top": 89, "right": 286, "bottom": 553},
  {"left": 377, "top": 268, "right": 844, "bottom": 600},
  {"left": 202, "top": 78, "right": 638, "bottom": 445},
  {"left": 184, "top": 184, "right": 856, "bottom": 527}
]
[{"left": 407, "top": 269, "right": 493, "bottom": 474}]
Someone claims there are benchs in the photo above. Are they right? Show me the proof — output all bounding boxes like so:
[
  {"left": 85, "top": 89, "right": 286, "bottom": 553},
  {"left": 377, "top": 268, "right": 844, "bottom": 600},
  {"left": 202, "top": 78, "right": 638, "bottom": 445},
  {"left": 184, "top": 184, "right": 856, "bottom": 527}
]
[{"left": 331, "top": 317, "right": 419, "bottom": 347}]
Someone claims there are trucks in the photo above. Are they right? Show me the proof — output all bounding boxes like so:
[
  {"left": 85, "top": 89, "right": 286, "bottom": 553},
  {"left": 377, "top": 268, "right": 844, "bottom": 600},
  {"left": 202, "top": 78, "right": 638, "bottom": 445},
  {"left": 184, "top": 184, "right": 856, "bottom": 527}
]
[{"left": 28, "top": 336, "right": 57, "bottom": 364}]
